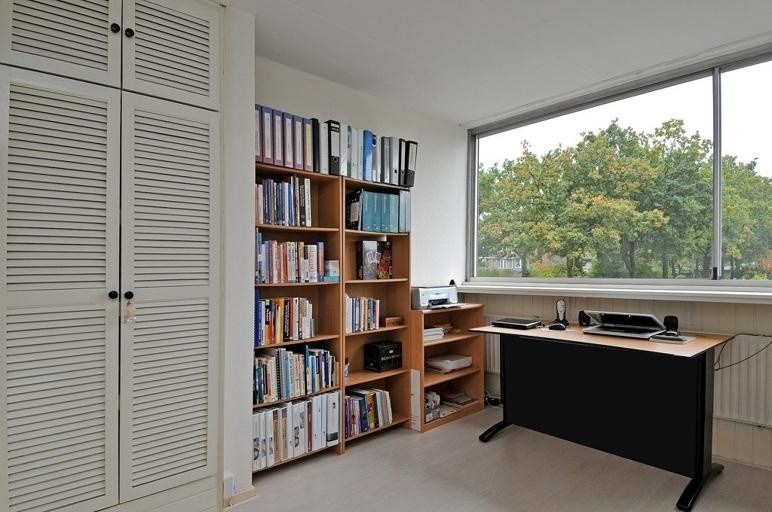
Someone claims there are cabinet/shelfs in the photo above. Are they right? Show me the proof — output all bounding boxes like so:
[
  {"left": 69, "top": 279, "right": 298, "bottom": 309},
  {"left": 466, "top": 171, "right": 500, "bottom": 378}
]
[
  {"left": 344, "top": 177, "right": 412, "bottom": 454},
  {"left": 411, "top": 303, "right": 486, "bottom": 433},
  {"left": 0, "top": 1, "right": 229, "bottom": 511},
  {"left": 253, "top": 162, "right": 344, "bottom": 475}
]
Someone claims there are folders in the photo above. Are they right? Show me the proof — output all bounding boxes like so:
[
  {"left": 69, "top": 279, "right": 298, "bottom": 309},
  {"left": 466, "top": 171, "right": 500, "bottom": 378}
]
[{"left": 254, "top": 104, "right": 418, "bottom": 187}]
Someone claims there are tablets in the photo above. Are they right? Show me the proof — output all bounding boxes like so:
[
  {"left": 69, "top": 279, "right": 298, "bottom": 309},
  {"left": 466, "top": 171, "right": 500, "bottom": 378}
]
[{"left": 492, "top": 317, "right": 539, "bottom": 329}]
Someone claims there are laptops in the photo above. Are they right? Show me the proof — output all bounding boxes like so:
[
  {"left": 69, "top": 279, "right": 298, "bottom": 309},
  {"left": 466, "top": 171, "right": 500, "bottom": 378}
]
[{"left": 583, "top": 310, "right": 666, "bottom": 340}]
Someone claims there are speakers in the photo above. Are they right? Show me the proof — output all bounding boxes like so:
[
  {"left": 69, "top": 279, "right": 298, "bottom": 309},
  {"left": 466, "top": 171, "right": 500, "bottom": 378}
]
[
  {"left": 664, "top": 316, "right": 678, "bottom": 336},
  {"left": 579, "top": 311, "right": 590, "bottom": 326}
]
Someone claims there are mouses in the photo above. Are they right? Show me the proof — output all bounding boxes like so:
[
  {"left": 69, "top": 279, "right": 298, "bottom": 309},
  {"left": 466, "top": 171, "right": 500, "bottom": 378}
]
[{"left": 549, "top": 323, "right": 566, "bottom": 330}]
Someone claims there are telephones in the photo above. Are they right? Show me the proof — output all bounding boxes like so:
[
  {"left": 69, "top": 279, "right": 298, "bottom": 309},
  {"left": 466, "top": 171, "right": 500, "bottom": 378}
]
[{"left": 547, "top": 298, "right": 569, "bottom": 329}]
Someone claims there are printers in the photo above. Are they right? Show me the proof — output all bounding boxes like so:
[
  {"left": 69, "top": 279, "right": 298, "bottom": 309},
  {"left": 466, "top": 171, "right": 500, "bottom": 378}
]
[{"left": 411, "top": 285, "right": 461, "bottom": 310}]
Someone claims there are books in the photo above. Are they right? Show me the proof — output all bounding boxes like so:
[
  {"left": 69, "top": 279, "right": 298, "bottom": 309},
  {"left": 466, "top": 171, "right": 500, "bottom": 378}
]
[
  {"left": 254, "top": 174, "right": 312, "bottom": 227},
  {"left": 355, "top": 241, "right": 392, "bottom": 279},
  {"left": 254, "top": 288, "right": 316, "bottom": 347},
  {"left": 346, "top": 295, "right": 400, "bottom": 333},
  {"left": 252, "top": 390, "right": 341, "bottom": 472},
  {"left": 253, "top": 344, "right": 339, "bottom": 404},
  {"left": 256, "top": 227, "right": 340, "bottom": 283},
  {"left": 253, "top": 104, "right": 418, "bottom": 187},
  {"left": 344, "top": 385, "right": 393, "bottom": 438},
  {"left": 347, "top": 191, "right": 412, "bottom": 233},
  {"left": 424, "top": 324, "right": 479, "bottom": 423}
]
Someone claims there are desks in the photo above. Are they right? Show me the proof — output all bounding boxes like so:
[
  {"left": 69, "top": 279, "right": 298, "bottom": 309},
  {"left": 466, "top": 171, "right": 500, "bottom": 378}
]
[{"left": 464, "top": 321, "right": 736, "bottom": 512}]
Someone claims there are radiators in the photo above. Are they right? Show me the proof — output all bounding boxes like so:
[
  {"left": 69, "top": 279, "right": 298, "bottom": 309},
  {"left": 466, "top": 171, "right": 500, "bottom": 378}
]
[{"left": 485, "top": 313, "right": 772, "bottom": 431}]
